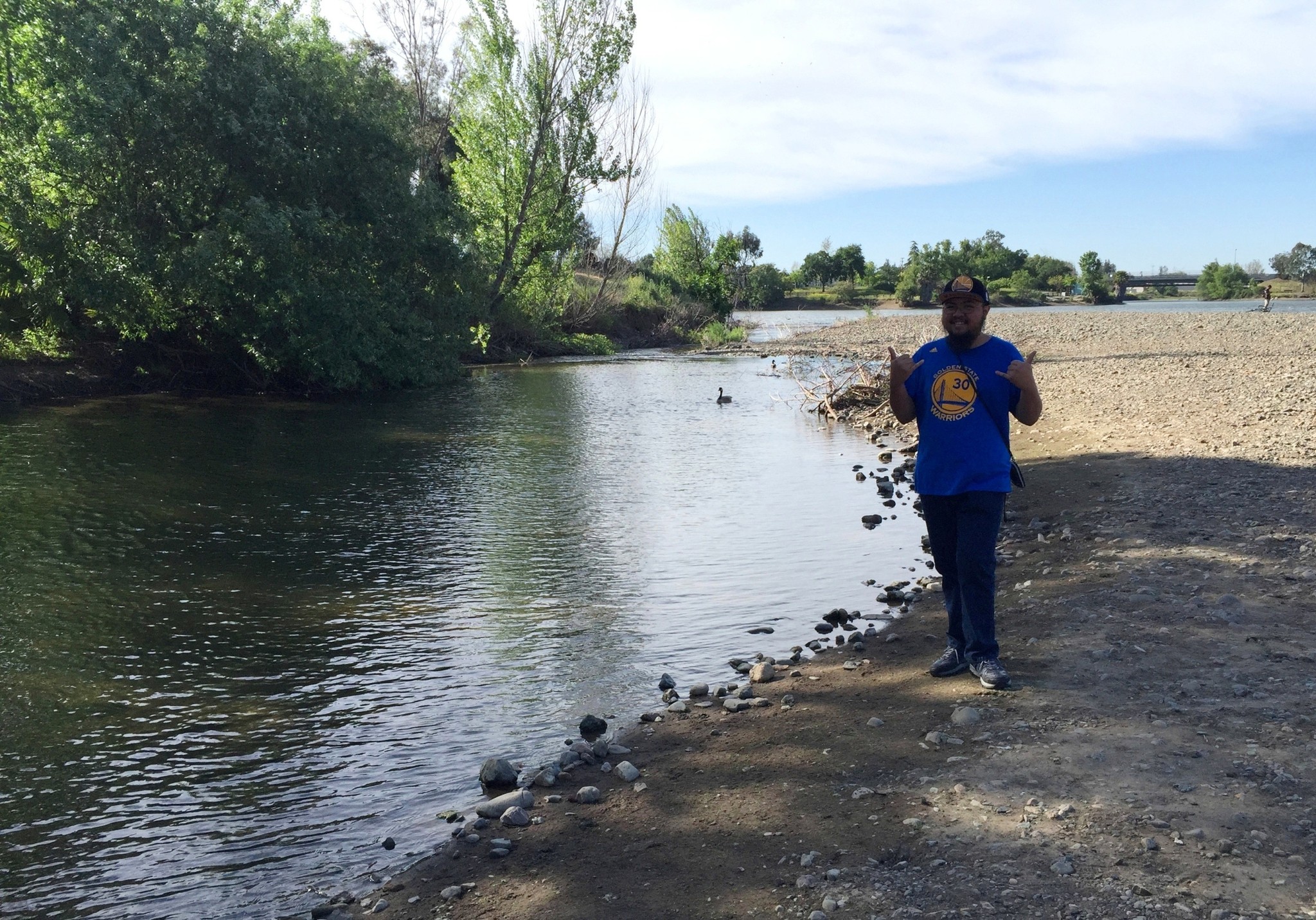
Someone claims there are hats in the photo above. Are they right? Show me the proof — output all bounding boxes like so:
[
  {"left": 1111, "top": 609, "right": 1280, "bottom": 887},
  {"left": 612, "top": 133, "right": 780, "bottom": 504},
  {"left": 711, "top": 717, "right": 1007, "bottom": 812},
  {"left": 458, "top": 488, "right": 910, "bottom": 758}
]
[{"left": 938, "top": 275, "right": 989, "bottom": 306}]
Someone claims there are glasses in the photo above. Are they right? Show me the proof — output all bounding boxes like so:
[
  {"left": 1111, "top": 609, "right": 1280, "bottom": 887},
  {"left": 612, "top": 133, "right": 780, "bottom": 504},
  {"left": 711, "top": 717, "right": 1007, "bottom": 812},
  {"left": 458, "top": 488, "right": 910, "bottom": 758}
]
[{"left": 943, "top": 303, "right": 984, "bottom": 314}]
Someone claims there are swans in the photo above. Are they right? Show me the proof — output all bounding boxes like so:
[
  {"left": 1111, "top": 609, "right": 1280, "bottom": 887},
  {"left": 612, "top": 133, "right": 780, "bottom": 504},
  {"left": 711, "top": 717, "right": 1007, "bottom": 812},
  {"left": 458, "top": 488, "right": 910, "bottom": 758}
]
[
  {"left": 715, "top": 386, "right": 733, "bottom": 405},
  {"left": 770, "top": 359, "right": 777, "bottom": 369}
]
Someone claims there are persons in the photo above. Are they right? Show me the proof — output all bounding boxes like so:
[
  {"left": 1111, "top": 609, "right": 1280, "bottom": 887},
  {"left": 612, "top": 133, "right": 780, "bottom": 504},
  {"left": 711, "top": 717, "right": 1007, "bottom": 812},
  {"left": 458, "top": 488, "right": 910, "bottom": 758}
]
[
  {"left": 884, "top": 277, "right": 1044, "bottom": 690},
  {"left": 1263, "top": 284, "right": 1273, "bottom": 312}
]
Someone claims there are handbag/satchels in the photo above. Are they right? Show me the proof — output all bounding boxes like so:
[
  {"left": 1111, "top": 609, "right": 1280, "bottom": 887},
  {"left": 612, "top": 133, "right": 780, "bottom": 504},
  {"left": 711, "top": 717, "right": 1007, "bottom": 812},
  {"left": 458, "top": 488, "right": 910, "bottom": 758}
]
[{"left": 1010, "top": 460, "right": 1026, "bottom": 489}]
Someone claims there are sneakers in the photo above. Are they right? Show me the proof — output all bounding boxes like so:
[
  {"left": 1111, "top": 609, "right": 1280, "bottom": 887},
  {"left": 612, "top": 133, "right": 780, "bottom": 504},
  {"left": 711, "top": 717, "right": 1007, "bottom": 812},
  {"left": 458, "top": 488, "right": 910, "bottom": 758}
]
[
  {"left": 929, "top": 646, "right": 968, "bottom": 676},
  {"left": 969, "top": 656, "right": 1012, "bottom": 690}
]
